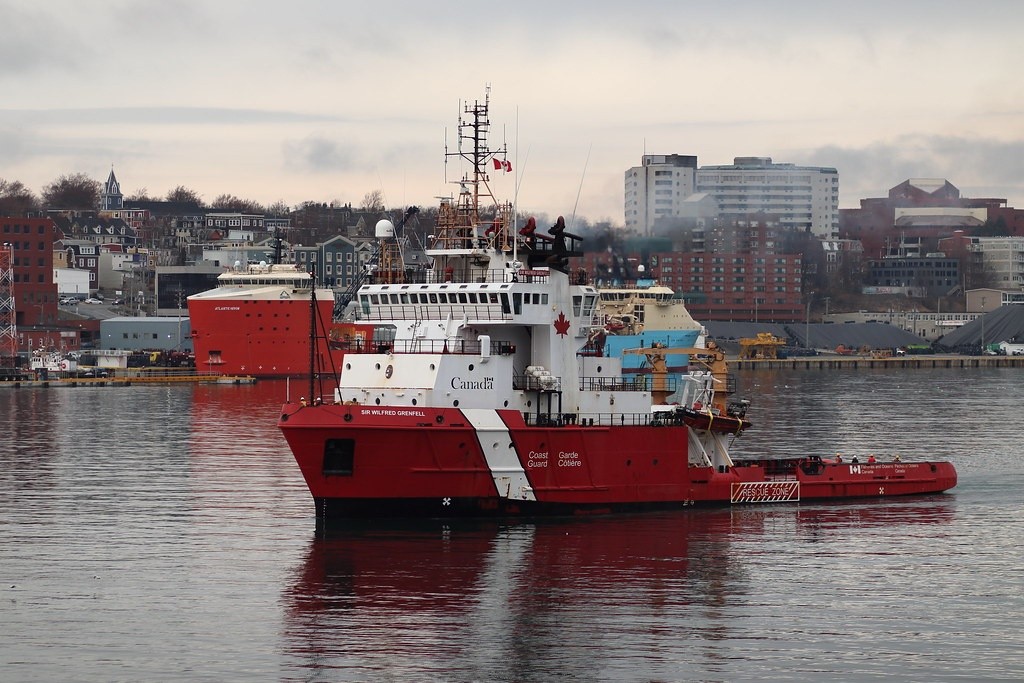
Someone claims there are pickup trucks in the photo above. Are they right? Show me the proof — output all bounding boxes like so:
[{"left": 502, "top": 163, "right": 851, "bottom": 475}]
[
  {"left": 59, "top": 297, "right": 80, "bottom": 306},
  {"left": 896, "top": 348, "right": 905, "bottom": 356},
  {"left": 1013, "top": 348, "right": 1024, "bottom": 356}
]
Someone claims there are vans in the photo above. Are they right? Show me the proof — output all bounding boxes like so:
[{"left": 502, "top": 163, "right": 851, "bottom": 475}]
[{"left": 91, "top": 292, "right": 104, "bottom": 300}]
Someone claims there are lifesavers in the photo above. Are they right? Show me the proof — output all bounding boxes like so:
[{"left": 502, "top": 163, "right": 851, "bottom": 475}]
[{"left": 61, "top": 363, "right": 66, "bottom": 369}]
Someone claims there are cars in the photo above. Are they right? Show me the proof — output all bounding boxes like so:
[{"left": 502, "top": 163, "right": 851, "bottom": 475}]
[
  {"left": 101, "top": 372, "right": 108, "bottom": 375},
  {"left": 59, "top": 294, "right": 67, "bottom": 301},
  {"left": 85, "top": 298, "right": 104, "bottom": 305},
  {"left": 112, "top": 299, "right": 125, "bottom": 306},
  {"left": 75, "top": 296, "right": 86, "bottom": 301},
  {"left": 984, "top": 349, "right": 997, "bottom": 355}
]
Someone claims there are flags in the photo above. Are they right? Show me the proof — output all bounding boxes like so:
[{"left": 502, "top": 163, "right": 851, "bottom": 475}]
[{"left": 493, "top": 158, "right": 512, "bottom": 172}]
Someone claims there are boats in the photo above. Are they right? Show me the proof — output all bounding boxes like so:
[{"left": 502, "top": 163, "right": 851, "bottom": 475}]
[
  {"left": 575, "top": 280, "right": 703, "bottom": 374},
  {"left": 186, "top": 225, "right": 394, "bottom": 380},
  {"left": 274, "top": 81, "right": 959, "bottom": 530}
]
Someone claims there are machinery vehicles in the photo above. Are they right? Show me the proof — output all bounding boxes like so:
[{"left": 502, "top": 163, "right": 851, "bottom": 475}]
[{"left": 149, "top": 351, "right": 188, "bottom": 366}]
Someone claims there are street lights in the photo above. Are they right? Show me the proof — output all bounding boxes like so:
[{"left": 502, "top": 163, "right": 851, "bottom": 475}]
[
  {"left": 981, "top": 305, "right": 984, "bottom": 356},
  {"left": 752, "top": 297, "right": 760, "bottom": 322},
  {"left": 806, "top": 299, "right": 813, "bottom": 349},
  {"left": 823, "top": 297, "right": 830, "bottom": 319}
]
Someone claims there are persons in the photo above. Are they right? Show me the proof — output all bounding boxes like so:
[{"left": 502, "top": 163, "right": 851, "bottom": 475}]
[
  {"left": 894, "top": 455, "right": 901, "bottom": 462},
  {"left": 300, "top": 397, "right": 306, "bottom": 406},
  {"left": 317, "top": 398, "right": 321, "bottom": 404},
  {"left": 851, "top": 455, "right": 858, "bottom": 463},
  {"left": 868, "top": 454, "right": 875, "bottom": 463},
  {"left": 836, "top": 453, "right": 842, "bottom": 463}
]
